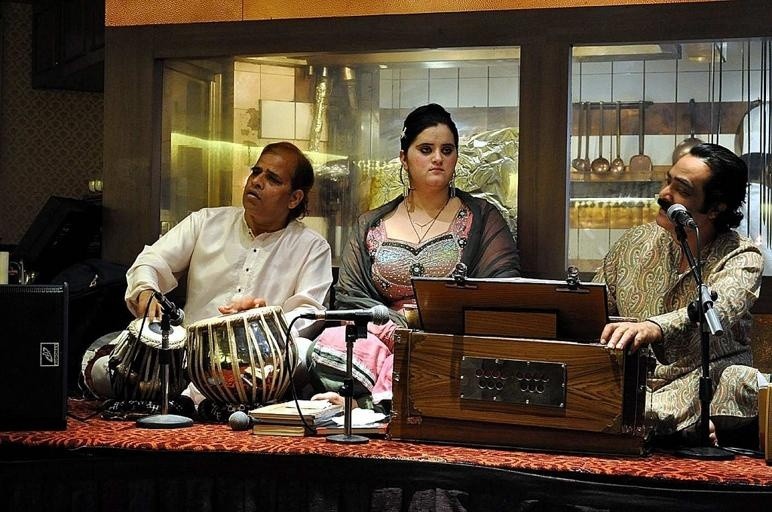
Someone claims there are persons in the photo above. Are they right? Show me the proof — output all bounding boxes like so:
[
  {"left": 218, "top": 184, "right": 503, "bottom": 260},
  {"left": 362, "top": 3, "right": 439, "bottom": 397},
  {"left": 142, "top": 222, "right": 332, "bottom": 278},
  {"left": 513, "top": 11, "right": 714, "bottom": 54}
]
[
  {"left": 90, "top": 141, "right": 334, "bottom": 424},
  {"left": 591, "top": 143, "right": 772, "bottom": 448},
  {"left": 305, "top": 103, "right": 521, "bottom": 423}
]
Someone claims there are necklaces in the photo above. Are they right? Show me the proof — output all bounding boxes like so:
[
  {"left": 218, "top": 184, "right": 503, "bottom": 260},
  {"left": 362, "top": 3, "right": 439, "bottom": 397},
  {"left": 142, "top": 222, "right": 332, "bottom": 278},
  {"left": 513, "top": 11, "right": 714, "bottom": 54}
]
[{"left": 406, "top": 198, "right": 449, "bottom": 243}]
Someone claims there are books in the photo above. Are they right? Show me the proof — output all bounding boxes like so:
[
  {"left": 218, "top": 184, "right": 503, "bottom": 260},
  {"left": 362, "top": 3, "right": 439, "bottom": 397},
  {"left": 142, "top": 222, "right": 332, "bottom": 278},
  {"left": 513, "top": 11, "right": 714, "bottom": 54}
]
[{"left": 248, "top": 399, "right": 388, "bottom": 437}]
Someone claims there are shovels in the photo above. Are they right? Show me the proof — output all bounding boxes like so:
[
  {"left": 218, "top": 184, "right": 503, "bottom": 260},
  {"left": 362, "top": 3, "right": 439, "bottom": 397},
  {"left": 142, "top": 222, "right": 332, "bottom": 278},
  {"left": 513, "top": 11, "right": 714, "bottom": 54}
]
[{"left": 629, "top": 101, "right": 653, "bottom": 179}]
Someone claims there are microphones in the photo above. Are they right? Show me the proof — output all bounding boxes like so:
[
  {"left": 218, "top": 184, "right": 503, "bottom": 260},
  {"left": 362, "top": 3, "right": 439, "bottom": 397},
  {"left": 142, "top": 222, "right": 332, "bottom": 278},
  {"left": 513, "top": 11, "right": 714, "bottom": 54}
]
[
  {"left": 666, "top": 203, "right": 698, "bottom": 231},
  {"left": 154, "top": 291, "right": 185, "bottom": 326},
  {"left": 228, "top": 410, "right": 252, "bottom": 430},
  {"left": 299, "top": 304, "right": 389, "bottom": 327}
]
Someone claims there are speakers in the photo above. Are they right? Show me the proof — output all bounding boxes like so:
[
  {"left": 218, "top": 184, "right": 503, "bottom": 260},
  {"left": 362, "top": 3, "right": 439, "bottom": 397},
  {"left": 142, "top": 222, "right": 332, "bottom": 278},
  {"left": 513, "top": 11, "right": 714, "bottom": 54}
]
[{"left": 0, "top": 282, "right": 70, "bottom": 431}]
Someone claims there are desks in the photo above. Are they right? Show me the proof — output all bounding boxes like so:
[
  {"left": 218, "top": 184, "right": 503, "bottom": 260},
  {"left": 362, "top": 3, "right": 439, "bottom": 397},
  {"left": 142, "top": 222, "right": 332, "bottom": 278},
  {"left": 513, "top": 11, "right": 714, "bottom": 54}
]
[{"left": 0, "top": 396, "right": 770, "bottom": 510}]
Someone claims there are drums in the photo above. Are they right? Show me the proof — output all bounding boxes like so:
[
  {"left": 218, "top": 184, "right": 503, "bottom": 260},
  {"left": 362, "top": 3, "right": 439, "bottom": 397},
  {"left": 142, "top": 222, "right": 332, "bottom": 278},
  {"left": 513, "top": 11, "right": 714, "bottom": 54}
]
[
  {"left": 108, "top": 317, "right": 190, "bottom": 400},
  {"left": 187, "top": 305, "right": 301, "bottom": 409}
]
